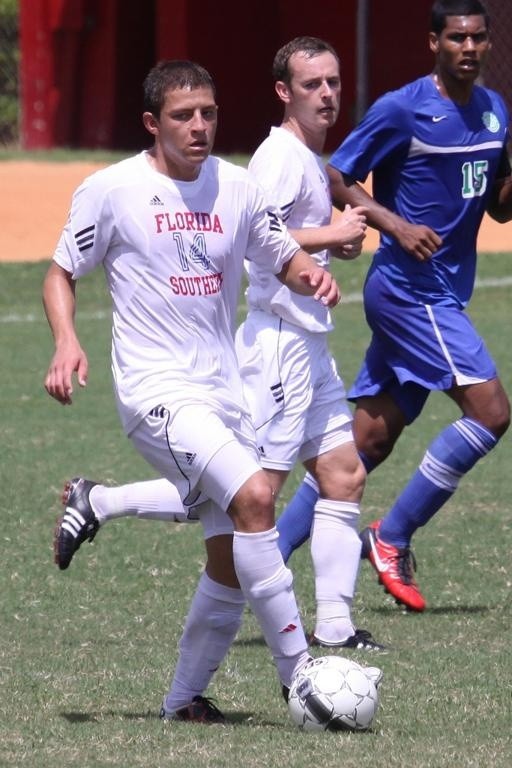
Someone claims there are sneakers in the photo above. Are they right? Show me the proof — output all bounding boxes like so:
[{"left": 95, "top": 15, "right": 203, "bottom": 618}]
[
  {"left": 53, "top": 478, "right": 103, "bottom": 570},
  {"left": 158, "top": 694, "right": 226, "bottom": 723},
  {"left": 284, "top": 668, "right": 383, "bottom": 713},
  {"left": 308, "top": 630, "right": 392, "bottom": 651},
  {"left": 359, "top": 518, "right": 424, "bottom": 611}
]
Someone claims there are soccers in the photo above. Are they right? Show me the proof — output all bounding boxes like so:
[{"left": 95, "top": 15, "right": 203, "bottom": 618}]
[{"left": 288, "top": 655, "right": 377, "bottom": 733}]
